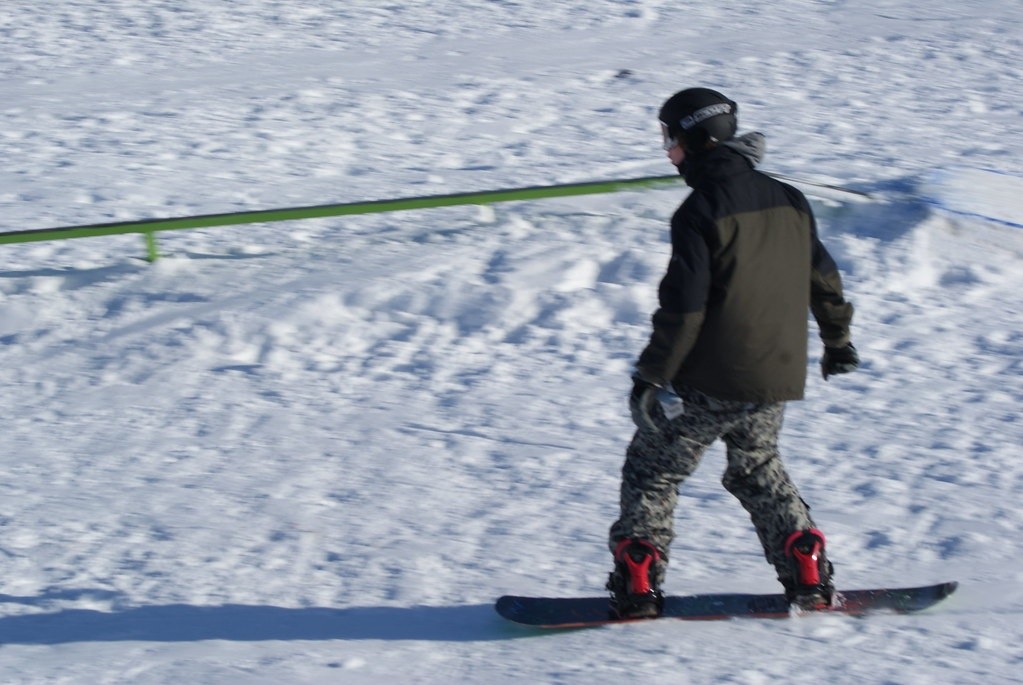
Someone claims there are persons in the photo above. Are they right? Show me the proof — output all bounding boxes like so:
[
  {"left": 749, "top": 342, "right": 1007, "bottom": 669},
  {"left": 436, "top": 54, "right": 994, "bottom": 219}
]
[{"left": 604, "top": 88, "right": 861, "bottom": 619}]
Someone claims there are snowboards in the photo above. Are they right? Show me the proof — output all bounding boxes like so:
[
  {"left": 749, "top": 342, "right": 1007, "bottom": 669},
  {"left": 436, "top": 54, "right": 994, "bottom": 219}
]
[{"left": 496, "top": 580, "right": 957, "bottom": 628}]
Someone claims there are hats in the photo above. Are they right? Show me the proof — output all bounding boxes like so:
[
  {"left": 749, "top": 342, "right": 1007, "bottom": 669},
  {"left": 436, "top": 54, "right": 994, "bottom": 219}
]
[{"left": 659, "top": 87, "right": 737, "bottom": 160}]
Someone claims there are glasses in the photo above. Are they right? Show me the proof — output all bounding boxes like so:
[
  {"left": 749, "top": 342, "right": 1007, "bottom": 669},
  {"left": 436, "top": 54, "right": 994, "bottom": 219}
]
[{"left": 661, "top": 137, "right": 679, "bottom": 151}]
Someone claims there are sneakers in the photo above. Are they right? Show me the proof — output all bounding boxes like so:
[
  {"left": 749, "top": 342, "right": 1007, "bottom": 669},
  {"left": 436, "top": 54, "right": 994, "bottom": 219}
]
[
  {"left": 778, "top": 529, "right": 833, "bottom": 606},
  {"left": 607, "top": 539, "right": 667, "bottom": 618}
]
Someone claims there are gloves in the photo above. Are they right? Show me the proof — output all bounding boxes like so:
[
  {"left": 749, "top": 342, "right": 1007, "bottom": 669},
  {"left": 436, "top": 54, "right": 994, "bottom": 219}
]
[
  {"left": 819, "top": 337, "right": 860, "bottom": 379},
  {"left": 631, "top": 380, "right": 670, "bottom": 433}
]
